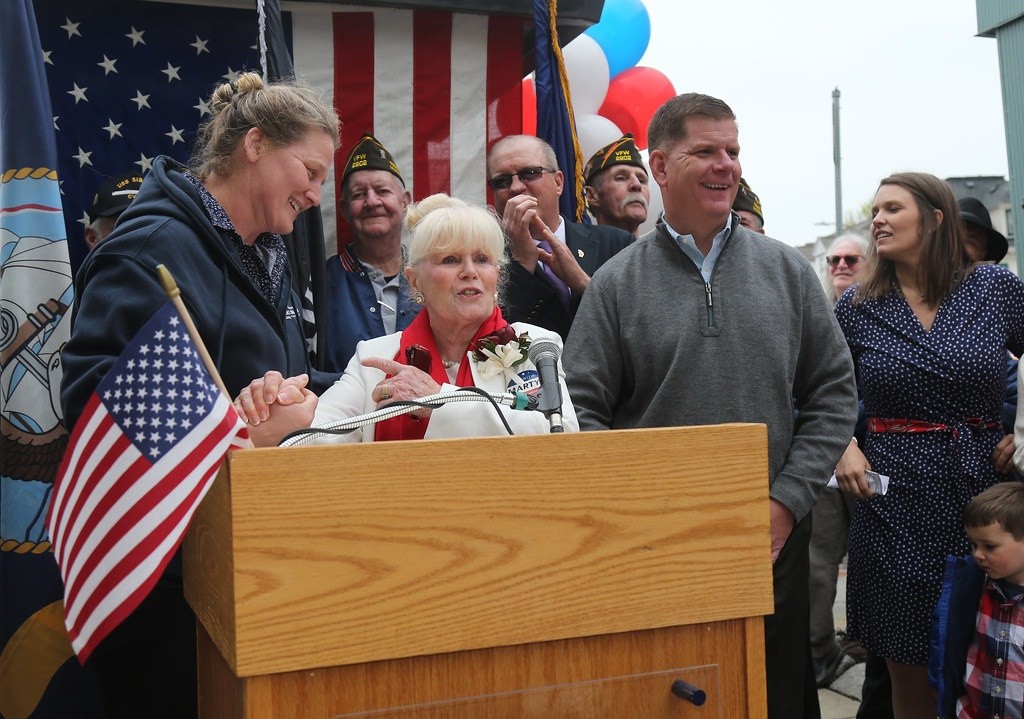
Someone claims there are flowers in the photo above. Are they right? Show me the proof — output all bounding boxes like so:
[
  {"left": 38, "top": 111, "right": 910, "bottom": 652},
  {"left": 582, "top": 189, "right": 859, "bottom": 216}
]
[{"left": 471, "top": 326, "right": 532, "bottom": 388}]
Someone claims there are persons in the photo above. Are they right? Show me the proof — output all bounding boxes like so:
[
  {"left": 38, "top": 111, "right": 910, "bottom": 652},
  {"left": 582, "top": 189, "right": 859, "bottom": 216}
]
[
  {"left": 732, "top": 178, "right": 765, "bottom": 234},
  {"left": 582, "top": 133, "right": 650, "bottom": 232},
  {"left": 235, "top": 193, "right": 580, "bottom": 446},
  {"left": 324, "top": 132, "right": 425, "bottom": 376},
  {"left": 927, "top": 482, "right": 1024, "bottom": 719},
  {"left": 487, "top": 135, "right": 638, "bottom": 340},
  {"left": 810, "top": 233, "right": 870, "bottom": 686},
  {"left": 561, "top": 93, "right": 859, "bottom": 719},
  {"left": 955, "top": 198, "right": 1008, "bottom": 264},
  {"left": 832, "top": 172, "right": 1024, "bottom": 719},
  {"left": 83, "top": 171, "right": 146, "bottom": 250},
  {"left": 57, "top": 72, "right": 340, "bottom": 719}
]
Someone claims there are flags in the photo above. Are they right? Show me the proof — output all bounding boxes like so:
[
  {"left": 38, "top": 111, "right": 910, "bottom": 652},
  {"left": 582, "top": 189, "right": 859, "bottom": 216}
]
[
  {"left": 44, "top": 299, "right": 250, "bottom": 665},
  {"left": 0, "top": 0, "right": 522, "bottom": 719}
]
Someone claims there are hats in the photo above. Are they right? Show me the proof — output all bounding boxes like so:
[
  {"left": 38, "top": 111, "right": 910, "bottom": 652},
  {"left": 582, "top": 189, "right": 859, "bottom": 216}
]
[
  {"left": 340, "top": 131, "right": 406, "bottom": 197},
  {"left": 92, "top": 171, "right": 144, "bottom": 216},
  {"left": 957, "top": 197, "right": 1008, "bottom": 265},
  {"left": 584, "top": 133, "right": 648, "bottom": 185},
  {"left": 732, "top": 178, "right": 763, "bottom": 226}
]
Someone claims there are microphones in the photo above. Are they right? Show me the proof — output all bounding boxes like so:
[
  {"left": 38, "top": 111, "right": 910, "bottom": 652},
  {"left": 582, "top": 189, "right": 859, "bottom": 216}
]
[{"left": 528, "top": 337, "right": 564, "bottom": 434}]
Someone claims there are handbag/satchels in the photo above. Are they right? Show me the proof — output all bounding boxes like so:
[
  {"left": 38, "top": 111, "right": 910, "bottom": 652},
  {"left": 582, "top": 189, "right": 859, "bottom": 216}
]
[{"left": 925, "top": 556, "right": 977, "bottom": 687}]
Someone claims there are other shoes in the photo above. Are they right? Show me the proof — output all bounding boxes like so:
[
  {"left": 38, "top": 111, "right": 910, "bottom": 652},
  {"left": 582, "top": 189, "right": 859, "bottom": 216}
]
[{"left": 812, "top": 642, "right": 843, "bottom": 688}]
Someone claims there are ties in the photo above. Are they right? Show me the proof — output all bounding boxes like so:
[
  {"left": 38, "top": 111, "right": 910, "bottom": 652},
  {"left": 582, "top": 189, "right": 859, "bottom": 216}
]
[{"left": 536, "top": 241, "right": 570, "bottom": 303}]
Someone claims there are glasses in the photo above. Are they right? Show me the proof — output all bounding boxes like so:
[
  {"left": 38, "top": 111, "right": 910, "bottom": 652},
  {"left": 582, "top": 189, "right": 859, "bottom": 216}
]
[
  {"left": 826, "top": 255, "right": 864, "bottom": 266},
  {"left": 487, "top": 166, "right": 555, "bottom": 189}
]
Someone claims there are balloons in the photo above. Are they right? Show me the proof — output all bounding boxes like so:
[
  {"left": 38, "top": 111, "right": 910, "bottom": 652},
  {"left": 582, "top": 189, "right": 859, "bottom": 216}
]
[{"left": 497, "top": 0, "right": 676, "bottom": 167}]
